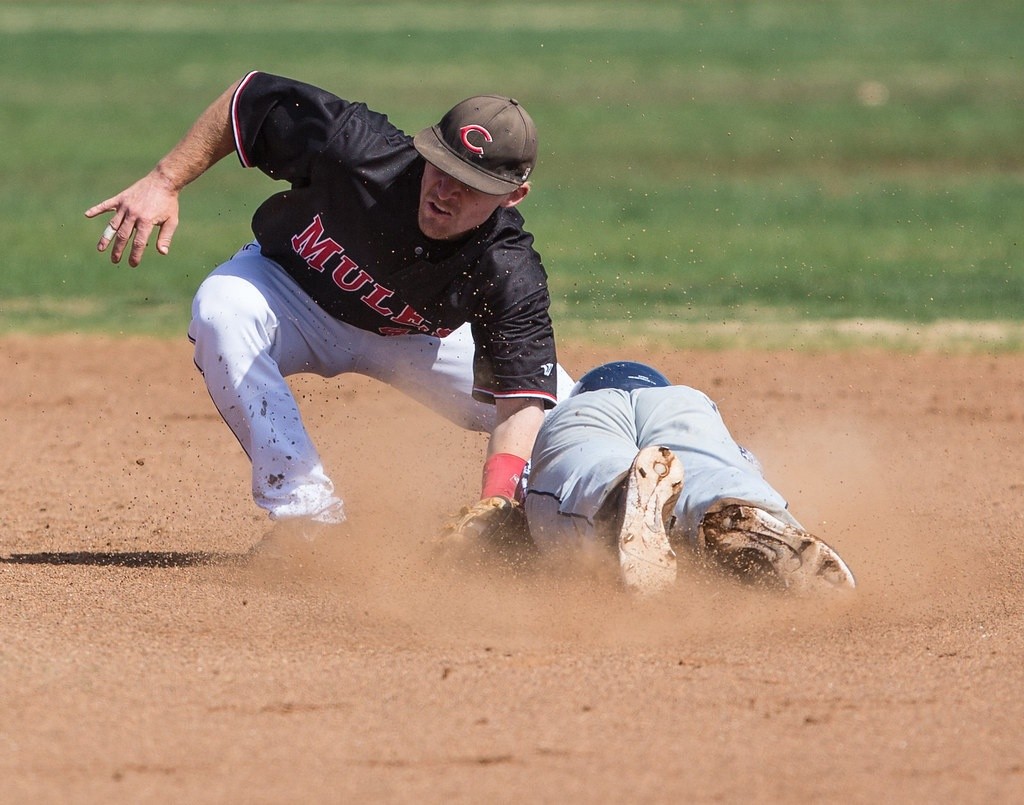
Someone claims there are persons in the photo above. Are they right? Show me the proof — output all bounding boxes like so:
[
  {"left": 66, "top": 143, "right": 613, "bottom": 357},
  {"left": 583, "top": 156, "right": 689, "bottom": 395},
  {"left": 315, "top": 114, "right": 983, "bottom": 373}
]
[
  {"left": 490, "top": 360, "right": 858, "bottom": 608},
  {"left": 81, "top": 67, "right": 575, "bottom": 578}
]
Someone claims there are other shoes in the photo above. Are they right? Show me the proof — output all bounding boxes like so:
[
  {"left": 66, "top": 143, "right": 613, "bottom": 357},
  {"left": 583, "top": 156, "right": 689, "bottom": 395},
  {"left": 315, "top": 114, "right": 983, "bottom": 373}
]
[
  {"left": 697, "top": 497, "right": 857, "bottom": 600},
  {"left": 242, "top": 518, "right": 352, "bottom": 574},
  {"left": 618, "top": 446, "right": 683, "bottom": 600}
]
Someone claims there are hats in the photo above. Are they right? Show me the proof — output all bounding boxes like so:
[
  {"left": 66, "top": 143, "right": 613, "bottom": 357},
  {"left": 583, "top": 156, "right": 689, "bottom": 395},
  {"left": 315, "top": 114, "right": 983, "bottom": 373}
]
[{"left": 413, "top": 94, "right": 538, "bottom": 195}]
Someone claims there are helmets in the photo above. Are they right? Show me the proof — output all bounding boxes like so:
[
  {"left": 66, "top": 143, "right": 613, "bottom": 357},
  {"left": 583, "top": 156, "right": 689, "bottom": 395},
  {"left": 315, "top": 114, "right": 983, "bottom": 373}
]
[{"left": 569, "top": 361, "right": 672, "bottom": 398}]
